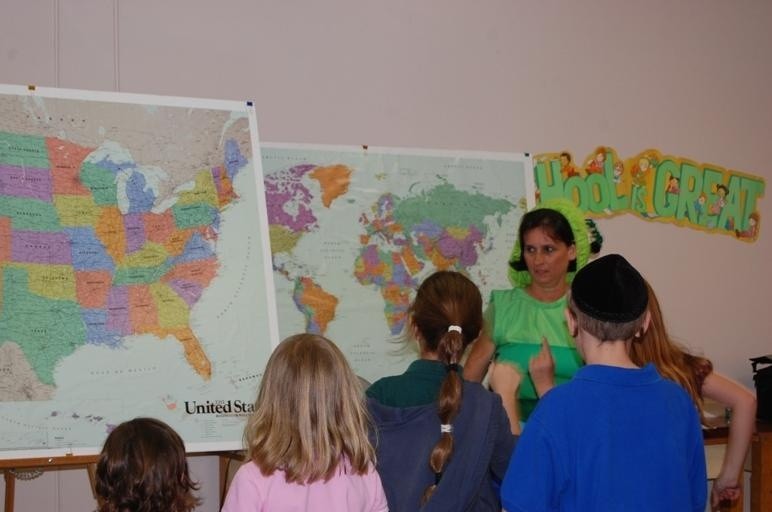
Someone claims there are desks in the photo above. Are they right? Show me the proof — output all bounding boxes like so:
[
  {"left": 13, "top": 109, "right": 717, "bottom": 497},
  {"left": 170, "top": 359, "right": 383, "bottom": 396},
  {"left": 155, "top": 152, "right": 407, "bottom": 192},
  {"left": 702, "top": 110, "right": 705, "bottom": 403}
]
[{"left": 704, "top": 427, "right": 772, "bottom": 512}]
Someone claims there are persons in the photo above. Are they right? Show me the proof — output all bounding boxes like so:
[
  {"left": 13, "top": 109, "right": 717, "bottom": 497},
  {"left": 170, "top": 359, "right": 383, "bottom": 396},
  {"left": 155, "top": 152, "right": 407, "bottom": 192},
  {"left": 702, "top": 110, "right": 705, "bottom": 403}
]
[
  {"left": 83, "top": 415, "right": 201, "bottom": 510},
  {"left": 363, "top": 272, "right": 518, "bottom": 510},
  {"left": 461, "top": 209, "right": 583, "bottom": 427},
  {"left": 628, "top": 281, "right": 757, "bottom": 510},
  {"left": 497, "top": 253, "right": 707, "bottom": 510},
  {"left": 216, "top": 332, "right": 389, "bottom": 512},
  {"left": 558, "top": 147, "right": 761, "bottom": 239}
]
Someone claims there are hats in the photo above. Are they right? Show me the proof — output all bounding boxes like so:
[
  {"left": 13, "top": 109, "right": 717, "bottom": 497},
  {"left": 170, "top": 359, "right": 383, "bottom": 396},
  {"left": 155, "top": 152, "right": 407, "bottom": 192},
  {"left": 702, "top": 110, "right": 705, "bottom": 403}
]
[{"left": 571, "top": 254, "right": 648, "bottom": 323}]
